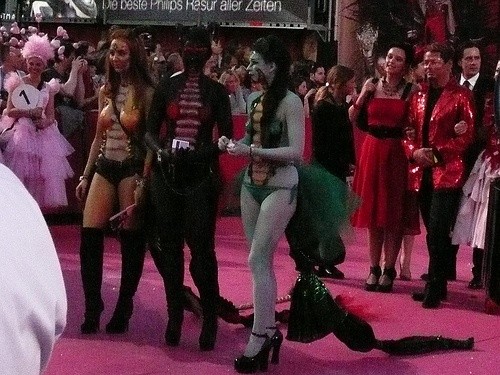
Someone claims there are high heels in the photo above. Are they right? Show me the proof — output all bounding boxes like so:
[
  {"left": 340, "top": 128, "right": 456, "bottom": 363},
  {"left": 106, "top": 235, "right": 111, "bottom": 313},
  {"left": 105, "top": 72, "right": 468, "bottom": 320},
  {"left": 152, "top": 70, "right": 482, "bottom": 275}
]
[
  {"left": 365, "top": 265, "right": 382, "bottom": 291},
  {"left": 376, "top": 266, "right": 397, "bottom": 292},
  {"left": 164, "top": 317, "right": 183, "bottom": 347},
  {"left": 233, "top": 327, "right": 284, "bottom": 373},
  {"left": 198, "top": 319, "right": 219, "bottom": 353}
]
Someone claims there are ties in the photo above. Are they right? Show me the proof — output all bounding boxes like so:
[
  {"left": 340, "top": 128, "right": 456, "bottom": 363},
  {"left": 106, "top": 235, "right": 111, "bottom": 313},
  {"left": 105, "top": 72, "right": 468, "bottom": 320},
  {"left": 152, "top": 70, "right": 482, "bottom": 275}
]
[{"left": 462, "top": 81, "right": 471, "bottom": 90}]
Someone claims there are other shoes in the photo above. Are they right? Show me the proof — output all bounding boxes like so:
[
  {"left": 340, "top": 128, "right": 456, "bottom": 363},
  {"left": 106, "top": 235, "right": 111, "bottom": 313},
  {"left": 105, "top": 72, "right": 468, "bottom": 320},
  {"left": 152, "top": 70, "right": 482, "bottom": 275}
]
[
  {"left": 421, "top": 284, "right": 442, "bottom": 308},
  {"left": 399, "top": 264, "right": 412, "bottom": 282},
  {"left": 299, "top": 261, "right": 344, "bottom": 283},
  {"left": 412, "top": 279, "right": 449, "bottom": 302},
  {"left": 485, "top": 296, "right": 500, "bottom": 316},
  {"left": 468, "top": 275, "right": 486, "bottom": 290},
  {"left": 421, "top": 268, "right": 457, "bottom": 282}
]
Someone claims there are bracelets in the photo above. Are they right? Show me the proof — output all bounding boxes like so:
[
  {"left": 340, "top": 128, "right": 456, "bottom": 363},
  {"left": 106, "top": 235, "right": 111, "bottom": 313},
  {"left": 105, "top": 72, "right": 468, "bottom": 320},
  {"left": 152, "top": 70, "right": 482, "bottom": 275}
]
[
  {"left": 27, "top": 108, "right": 33, "bottom": 117},
  {"left": 250, "top": 147, "right": 253, "bottom": 156},
  {"left": 78, "top": 175, "right": 88, "bottom": 181}
]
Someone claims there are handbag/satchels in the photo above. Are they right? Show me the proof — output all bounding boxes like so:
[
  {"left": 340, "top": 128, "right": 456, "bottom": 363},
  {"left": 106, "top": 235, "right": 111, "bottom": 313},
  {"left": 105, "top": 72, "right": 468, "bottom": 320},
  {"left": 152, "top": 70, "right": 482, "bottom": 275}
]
[{"left": 355, "top": 101, "right": 369, "bottom": 133}]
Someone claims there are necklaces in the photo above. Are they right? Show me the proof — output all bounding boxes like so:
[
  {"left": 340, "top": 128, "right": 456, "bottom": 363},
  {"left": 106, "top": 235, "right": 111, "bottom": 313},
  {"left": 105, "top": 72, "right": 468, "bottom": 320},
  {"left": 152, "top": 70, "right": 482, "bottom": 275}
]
[{"left": 381, "top": 74, "right": 405, "bottom": 97}]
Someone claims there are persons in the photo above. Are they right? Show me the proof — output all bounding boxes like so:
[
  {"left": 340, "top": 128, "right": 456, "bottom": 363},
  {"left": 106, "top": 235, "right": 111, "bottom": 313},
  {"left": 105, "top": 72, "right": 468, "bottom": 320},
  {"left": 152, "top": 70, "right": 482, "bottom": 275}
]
[
  {"left": 1, "top": 163, "right": 67, "bottom": 375},
  {"left": 73, "top": 27, "right": 158, "bottom": 337},
  {"left": 219, "top": 37, "right": 307, "bottom": 372},
  {"left": 346, "top": 43, "right": 425, "bottom": 294},
  {"left": 0, "top": 0, "right": 500, "bottom": 375}
]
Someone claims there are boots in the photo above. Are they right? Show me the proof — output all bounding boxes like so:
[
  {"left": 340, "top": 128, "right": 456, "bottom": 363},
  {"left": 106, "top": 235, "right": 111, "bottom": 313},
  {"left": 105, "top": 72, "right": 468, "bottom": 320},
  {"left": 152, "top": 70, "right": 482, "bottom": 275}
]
[
  {"left": 79, "top": 225, "right": 104, "bottom": 334},
  {"left": 105, "top": 227, "right": 150, "bottom": 334}
]
